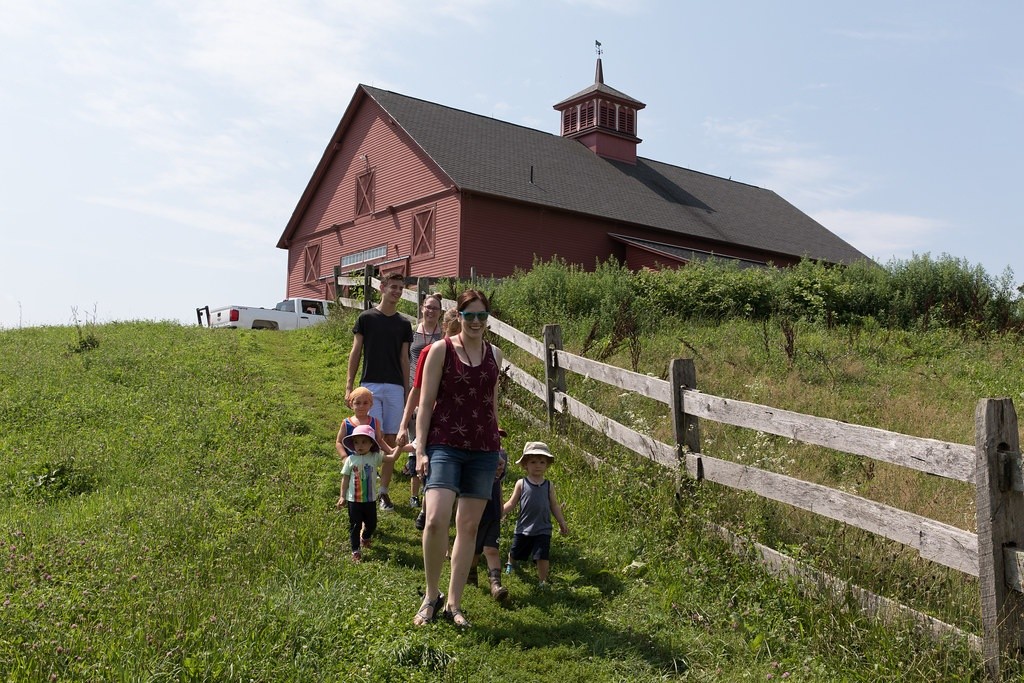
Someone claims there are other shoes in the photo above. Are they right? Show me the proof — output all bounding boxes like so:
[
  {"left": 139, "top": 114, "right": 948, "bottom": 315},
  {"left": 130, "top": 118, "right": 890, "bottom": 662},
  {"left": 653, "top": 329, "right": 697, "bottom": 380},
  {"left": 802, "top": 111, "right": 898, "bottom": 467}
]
[
  {"left": 415, "top": 511, "right": 426, "bottom": 530},
  {"left": 410, "top": 497, "right": 421, "bottom": 509},
  {"left": 361, "top": 533, "right": 371, "bottom": 548},
  {"left": 352, "top": 551, "right": 363, "bottom": 564}
]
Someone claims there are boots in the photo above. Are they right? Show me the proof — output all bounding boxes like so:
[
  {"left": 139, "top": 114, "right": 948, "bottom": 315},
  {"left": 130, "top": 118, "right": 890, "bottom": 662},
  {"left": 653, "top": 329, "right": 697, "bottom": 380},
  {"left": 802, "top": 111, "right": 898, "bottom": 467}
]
[
  {"left": 467, "top": 567, "right": 478, "bottom": 588},
  {"left": 489, "top": 568, "right": 508, "bottom": 601}
]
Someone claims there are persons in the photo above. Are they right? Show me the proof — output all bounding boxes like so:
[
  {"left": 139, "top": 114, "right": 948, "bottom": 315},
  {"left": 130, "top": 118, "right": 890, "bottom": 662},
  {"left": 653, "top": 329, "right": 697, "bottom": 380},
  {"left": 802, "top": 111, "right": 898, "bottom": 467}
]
[
  {"left": 398, "top": 309, "right": 511, "bottom": 603},
  {"left": 330, "top": 384, "right": 408, "bottom": 562},
  {"left": 414, "top": 289, "right": 504, "bottom": 630},
  {"left": 410, "top": 293, "right": 443, "bottom": 507},
  {"left": 501, "top": 441, "right": 568, "bottom": 595},
  {"left": 344, "top": 273, "right": 413, "bottom": 512}
]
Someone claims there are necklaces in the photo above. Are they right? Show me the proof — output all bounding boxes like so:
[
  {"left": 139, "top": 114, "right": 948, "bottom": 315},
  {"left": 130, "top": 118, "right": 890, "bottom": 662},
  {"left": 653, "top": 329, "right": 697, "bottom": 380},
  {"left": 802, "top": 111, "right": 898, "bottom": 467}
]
[
  {"left": 420, "top": 322, "right": 439, "bottom": 346},
  {"left": 458, "top": 333, "right": 485, "bottom": 367}
]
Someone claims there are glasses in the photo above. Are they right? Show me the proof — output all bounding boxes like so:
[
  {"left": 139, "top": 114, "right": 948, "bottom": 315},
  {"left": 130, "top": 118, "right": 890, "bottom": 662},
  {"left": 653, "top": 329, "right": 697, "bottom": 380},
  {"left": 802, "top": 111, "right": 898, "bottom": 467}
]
[
  {"left": 424, "top": 306, "right": 441, "bottom": 312},
  {"left": 458, "top": 311, "right": 489, "bottom": 322}
]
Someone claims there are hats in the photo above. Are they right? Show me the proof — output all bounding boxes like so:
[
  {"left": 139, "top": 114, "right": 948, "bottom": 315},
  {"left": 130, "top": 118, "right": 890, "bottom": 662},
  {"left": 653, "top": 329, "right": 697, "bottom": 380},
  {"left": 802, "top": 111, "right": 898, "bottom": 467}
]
[
  {"left": 342, "top": 425, "right": 380, "bottom": 453},
  {"left": 515, "top": 442, "right": 554, "bottom": 468},
  {"left": 497, "top": 427, "right": 508, "bottom": 439}
]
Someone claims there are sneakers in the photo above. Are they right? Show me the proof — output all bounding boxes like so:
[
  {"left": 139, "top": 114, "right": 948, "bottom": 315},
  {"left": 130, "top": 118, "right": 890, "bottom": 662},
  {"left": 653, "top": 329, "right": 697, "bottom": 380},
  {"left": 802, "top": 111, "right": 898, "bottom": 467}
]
[{"left": 377, "top": 492, "right": 395, "bottom": 510}]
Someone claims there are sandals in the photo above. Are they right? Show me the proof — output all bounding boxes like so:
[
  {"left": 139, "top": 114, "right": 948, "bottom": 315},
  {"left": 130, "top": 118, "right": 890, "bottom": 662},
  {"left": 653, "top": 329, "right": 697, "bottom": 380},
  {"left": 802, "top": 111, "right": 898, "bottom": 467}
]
[
  {"left": 443, "top": 606, "right": 472, "bottom": 628},
  {"left": 414, "top": 589, "right": 445, "bottom": 627}
]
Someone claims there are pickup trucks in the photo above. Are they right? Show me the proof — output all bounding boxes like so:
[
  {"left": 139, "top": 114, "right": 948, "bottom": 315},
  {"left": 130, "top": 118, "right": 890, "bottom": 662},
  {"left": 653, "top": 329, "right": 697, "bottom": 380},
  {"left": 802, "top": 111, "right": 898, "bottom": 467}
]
[{"left": 196, "top": 297, "right": 340, "bottom": 332}]
